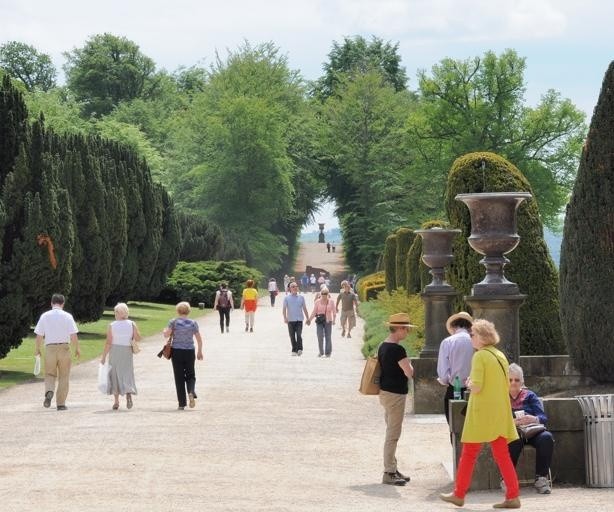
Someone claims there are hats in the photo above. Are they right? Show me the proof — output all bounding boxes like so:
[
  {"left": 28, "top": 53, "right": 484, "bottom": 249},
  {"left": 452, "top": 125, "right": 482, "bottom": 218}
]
[
  {"left": 446, "top": 311, "right": 474, "bottom": 335},
  {"left": 383, "top": 313, "right": 418, "bottom": 327}
]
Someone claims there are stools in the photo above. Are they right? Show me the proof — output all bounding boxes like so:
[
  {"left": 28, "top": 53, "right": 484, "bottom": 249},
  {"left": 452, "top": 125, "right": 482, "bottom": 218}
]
[{"left": 515, "top": 443, "right": 554, "bottom": 492}]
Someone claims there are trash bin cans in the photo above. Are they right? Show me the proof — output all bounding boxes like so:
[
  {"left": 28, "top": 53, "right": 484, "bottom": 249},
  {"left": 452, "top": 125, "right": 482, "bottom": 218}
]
[{"left": 574, "top": 394, "right": 614, "bottom": 488}]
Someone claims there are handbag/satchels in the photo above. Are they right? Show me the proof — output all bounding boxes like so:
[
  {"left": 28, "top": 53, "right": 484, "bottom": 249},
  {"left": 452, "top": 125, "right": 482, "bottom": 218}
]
[
  {"left": 163, "top": 344, "right": 172, "bottom": 360},
  {"left": 359, "top": 357, "right": 382, "bottom": 395},
  {"left": 315, "top": 314, "right": 325, "bottom": 324},
  {"left": 131, "top": 338, "right": 141, "bottom": 354},
  {"left": 518, "top": 423, "right": 547, "bottom": 440}
]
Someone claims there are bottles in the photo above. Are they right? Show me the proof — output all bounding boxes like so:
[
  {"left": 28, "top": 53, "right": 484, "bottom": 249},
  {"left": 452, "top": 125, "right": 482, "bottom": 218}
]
[{"left": 453, "top": 375, "right": 462, "bottom": 399}]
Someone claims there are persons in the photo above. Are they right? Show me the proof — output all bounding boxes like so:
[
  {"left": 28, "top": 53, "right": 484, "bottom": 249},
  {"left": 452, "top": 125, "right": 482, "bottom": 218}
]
[
  {"left": 162, "top": 301, "right": 203, "bottom": 410},
  {"left": 240, "top": 280, "right": 259, "bottom": 332},
  {"left": 214, "top": 282, "right": 234, "bottom": 334},
  {"left": 438, "top": 318, "right": 521, "bottom": 509},
  {"left": 500, "top": 362, "right": 554, "bottom": 494},
  {"left": 283, "top": 282, "right": 310, "bottom": 357},
  {"left": 101, "top": 302, "right": 140, "bottom": 410},
  {"left": 33, "top": 293, "right": 81, "bottom": 410},
  {"left": 268, "top": 277, "right": 277, "bottom": 307},
  {"left": 336, "top": 285, "right": 358, "bottom": 338},
  {"left": 378, "top": 312, "right": 418, "bottom": 486},
  {"left": 283, "top": 272, "right": 331, "bottom": 303},
  {"left": 340, "top": 280, "right": 353, "bottom": 293},
  {"left": 327, "top": 242, "right": 330, "bottom": 252},
  {"left": 308, "top": 288, "right": 336, "bottom": 358},
  {"left": 332, "top": 241, "right": 335, "bottom": 252},
  {"left": 436, "top": 312, "right": 473, "bottom": 425}
]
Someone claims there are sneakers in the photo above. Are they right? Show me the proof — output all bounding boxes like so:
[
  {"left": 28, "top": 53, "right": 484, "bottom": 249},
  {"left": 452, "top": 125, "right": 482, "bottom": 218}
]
[
  {"left": 439, "top": 493, "right": 464, "bottom": 506},
  {"left": 383, "top": 471, "right": 409, "bottom": 486},
  {"left": 189, "top": 393, "right": 195, "bottom": 408},
  {"left": 44, "top": 391, "right": 53, "bottom": 408},
  {"left": 493, "top": 498, "right": 520, "bottom": 509},
  {"left": 535, "top": 479, "right": 552, "bottom": 494}
]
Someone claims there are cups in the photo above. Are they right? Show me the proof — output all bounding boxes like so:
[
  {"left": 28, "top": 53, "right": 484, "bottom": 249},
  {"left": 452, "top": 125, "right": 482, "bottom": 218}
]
[{"left": 513, "top": 409, "right": 525, "bottom": 417}]
